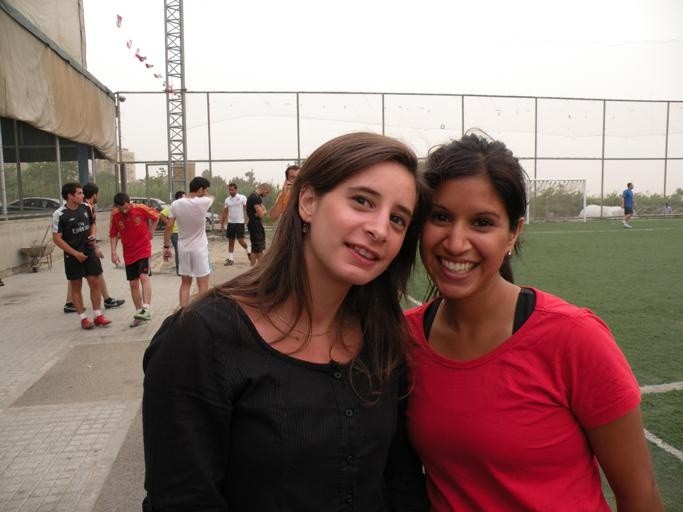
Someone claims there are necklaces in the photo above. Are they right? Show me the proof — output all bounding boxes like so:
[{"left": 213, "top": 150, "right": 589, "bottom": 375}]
[{"left": 271, "top": 294, "right": 337, "bottom": 337}]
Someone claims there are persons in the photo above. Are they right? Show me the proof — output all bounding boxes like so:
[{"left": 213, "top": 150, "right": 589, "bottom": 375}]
[
  {"left": 63, "top": 183, "right": 125, "bottom": 313},
  {"left": 397, "top": 134, "right": 664, "bottom": 511},
  {"left": 268, "top": 166, "right": 300, "bottom": 222},
  {"left": 50, "top": 183, "right": 110, "bottom": 329},
  {"left": 661, "top": 203, "right": 672, "bottom": 214},
  {"left": 159, "top": 191, "right": 186, "bottom": 276},
  {"left": 220, "top": 183, "right": 251, "bottom": 266},
  {"left": 142, "top": 130, "right": 424, "bottom": 511},
  {"left": 245, "top": 183, "right": 271, "bottom": 267},
  {"left": 621, "top": 182, "right": 634, "bottom": 228},
  {"left": 162, "top": 177, "right": 214, "bottom": 310},
  {"left": 109, "top": 193, "right": 159, "bottom": 329}
]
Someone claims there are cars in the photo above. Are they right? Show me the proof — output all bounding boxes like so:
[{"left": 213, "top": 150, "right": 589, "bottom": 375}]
[
  {"left": 206, "top": 211, "right": 220, "bottom": 226},
  {"left": 1, "top": 194, "right": 64, "bottom": 211}
]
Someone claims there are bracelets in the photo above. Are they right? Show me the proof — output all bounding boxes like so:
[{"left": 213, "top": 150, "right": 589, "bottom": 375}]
[{"left": 163, "top": 245, "right": 170, "bottom": 249}]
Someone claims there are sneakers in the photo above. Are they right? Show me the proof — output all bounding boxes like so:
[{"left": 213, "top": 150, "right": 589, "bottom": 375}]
[
  {"left": 248, "top": 253, "right": 251, "bottom": 260},
  {"left": 224, "top": 259, "right": 234, "bottom": 266},
  {"left": 62, "top": 297, "right": 153, "bottom": 329},
  {"left": 621, "top": 220, "right": 626, "bottom": 223},
  {"left": 625, "top": 225, "right": 631, "bottom": 228}
]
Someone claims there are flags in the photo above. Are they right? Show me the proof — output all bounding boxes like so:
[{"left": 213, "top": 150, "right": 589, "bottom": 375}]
[{"left": 116, "top": 15, "right": 181, "bottom": 98}]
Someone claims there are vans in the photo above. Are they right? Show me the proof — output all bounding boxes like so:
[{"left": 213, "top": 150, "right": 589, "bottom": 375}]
[{"left": 130, "top": 197, "right": 170, "bottom": 211}]
